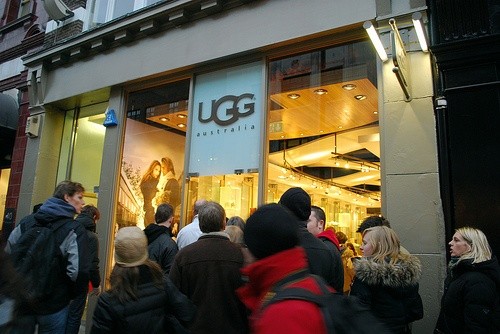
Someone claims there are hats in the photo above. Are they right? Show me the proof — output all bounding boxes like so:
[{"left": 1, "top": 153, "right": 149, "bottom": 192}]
[
  {"left": 114, "top": 226, "right": 149, "bottom": 267},
  {"left": 244, "top": 203, "right": 301, "bottom": 260},
  {"left": 280, "top": 187, "right": 311, "bottom": 222}
]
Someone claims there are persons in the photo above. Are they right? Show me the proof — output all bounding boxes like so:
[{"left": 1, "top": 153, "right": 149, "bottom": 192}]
[
  {"left": 91, "top": 187, "right": 424, "bottom": 334},
  {"left": 141, "top": 156, "right": 180, "bottom": 229},
  {"left": 1, "top": 180, "right": 101, "bottom": 334},
  {"left": 433, "top": 225, "right": 500, "bottom": 334}
]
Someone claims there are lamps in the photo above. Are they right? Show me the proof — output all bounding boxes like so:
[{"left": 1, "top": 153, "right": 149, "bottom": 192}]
[
  {"left": 363, "top": 21, "right": 389, "bottom": 63},
  {"left": 412, "top": 12, "right": 430, "bottom": 52},
  {"left": 43, "top": 0, "right": 74, "bottom": 22}
]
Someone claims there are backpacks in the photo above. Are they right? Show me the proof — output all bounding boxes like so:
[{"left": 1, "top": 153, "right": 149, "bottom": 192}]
[
  {"left": 264, "top": 274, "right": 391, "bottom": 334},
  {"left": 4, "top": 213, "right": 76, "bottom": 311}
]
